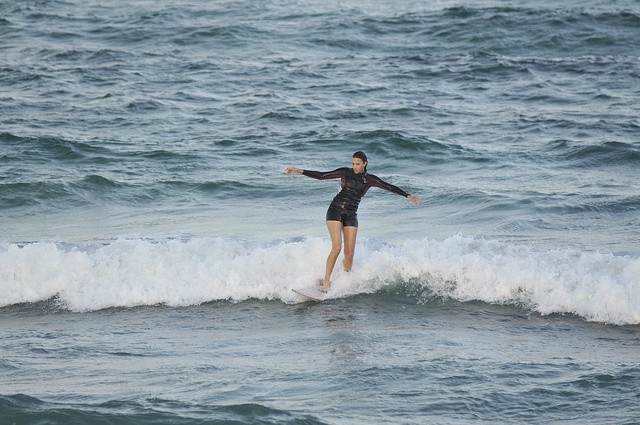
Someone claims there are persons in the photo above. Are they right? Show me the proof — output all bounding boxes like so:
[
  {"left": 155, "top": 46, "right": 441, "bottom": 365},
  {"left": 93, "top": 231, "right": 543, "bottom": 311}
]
[{"left": 284, "top": 150, "right": 422, "bottom": 288}]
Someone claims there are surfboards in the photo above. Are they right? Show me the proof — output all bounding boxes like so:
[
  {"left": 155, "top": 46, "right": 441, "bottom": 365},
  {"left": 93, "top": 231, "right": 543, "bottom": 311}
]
[{"left": 292, "top": 285, "right": 328, "bottom": 301}]
